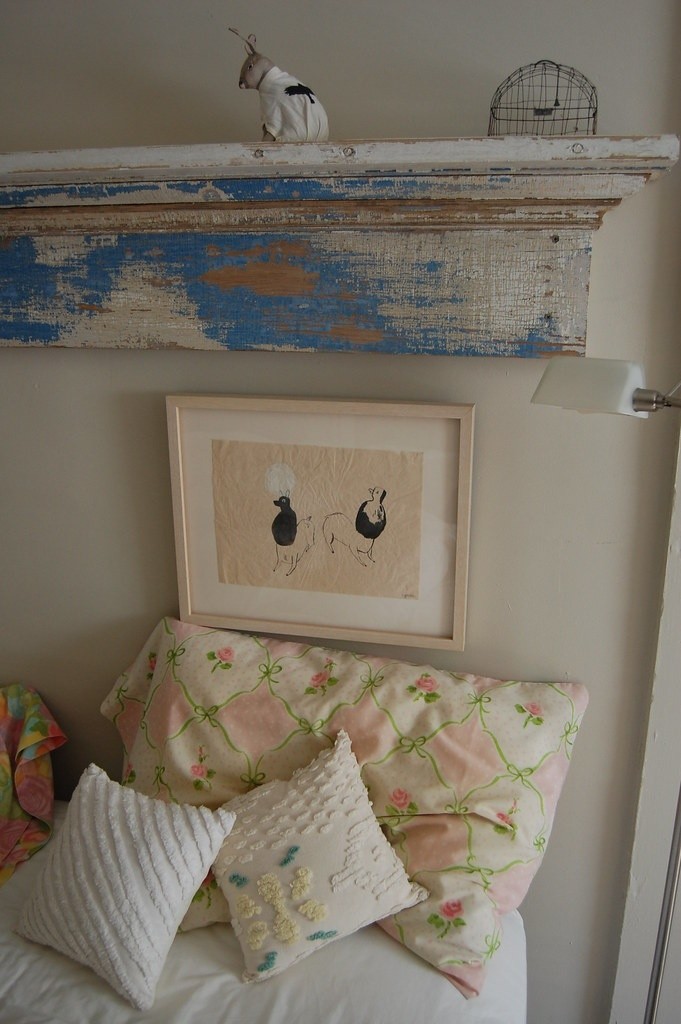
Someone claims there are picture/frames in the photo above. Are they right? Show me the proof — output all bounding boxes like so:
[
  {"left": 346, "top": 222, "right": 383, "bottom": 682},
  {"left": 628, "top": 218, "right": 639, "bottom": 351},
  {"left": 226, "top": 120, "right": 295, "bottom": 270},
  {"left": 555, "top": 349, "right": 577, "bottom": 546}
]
[{"left": 164, "top": 392, "right": 474, "bottom": 651}]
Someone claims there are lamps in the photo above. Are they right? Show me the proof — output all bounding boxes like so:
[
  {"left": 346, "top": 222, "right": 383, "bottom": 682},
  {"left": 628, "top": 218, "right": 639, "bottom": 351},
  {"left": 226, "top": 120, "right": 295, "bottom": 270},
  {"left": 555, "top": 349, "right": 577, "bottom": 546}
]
[{"left": 532, "top": 355, "right": 681, "bottom": 420}]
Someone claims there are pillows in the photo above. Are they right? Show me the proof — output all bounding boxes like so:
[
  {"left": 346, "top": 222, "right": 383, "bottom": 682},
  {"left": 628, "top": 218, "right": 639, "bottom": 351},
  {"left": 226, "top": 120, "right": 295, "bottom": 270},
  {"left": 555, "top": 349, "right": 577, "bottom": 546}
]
[
  {"left": 209, "top": 728, "right": 430, "bottom": 984},
  {"left": 9, "top": 763, "right": 236, "bottom": 1010},
  {"left": 99, "top": 616, "right": 588, "bottom": 1000}
]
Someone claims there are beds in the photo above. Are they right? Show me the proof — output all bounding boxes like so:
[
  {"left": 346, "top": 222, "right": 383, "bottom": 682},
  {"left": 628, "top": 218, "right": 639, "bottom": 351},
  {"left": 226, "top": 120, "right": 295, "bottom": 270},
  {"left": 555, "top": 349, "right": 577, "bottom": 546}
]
[{"left": 0, "top": 802, "right": 527, "bottom": 1024}]
